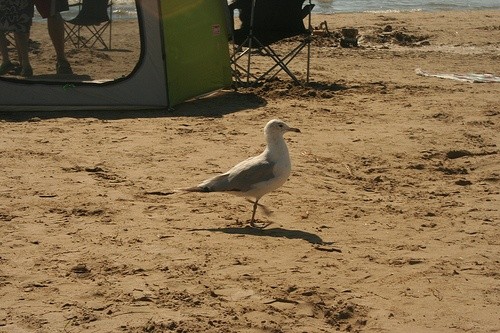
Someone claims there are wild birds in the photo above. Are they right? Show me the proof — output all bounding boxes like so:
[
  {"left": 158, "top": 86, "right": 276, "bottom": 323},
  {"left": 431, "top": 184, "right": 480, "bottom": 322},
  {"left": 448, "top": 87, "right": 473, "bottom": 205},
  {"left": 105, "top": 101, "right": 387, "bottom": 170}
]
[{"left": 178, "top": 118, "right": 304, "bottom": 228}]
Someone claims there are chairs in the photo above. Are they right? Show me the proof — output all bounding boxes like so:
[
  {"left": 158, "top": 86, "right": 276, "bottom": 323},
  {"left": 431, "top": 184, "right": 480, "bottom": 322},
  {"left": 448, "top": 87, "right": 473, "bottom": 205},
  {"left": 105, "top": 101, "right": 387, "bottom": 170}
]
[
  {"left": 59, "top": 0, "right": 115, "bottom": 51},
  {"left": 226, "top": 0, "right": 315, "bottom": 89}
]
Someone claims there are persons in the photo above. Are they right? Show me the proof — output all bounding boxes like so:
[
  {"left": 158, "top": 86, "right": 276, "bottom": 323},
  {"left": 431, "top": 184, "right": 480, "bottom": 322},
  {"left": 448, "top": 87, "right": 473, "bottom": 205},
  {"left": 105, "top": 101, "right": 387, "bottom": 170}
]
[{"left": 0, "top": 1, "right": 75, "bottom": 82}]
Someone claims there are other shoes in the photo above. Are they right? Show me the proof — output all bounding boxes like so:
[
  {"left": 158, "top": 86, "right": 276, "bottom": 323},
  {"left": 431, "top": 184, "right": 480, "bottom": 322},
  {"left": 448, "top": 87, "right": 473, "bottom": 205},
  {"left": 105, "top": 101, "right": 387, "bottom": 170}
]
[
  {"left": 56, "top": 61, "right": 73, "bottom": 74},
  {"left": 19, "top": 62, "right": 33, "bottom": 76},
  {"left": 0, "top": 61, "right": 15, "bottom": 76}
]
